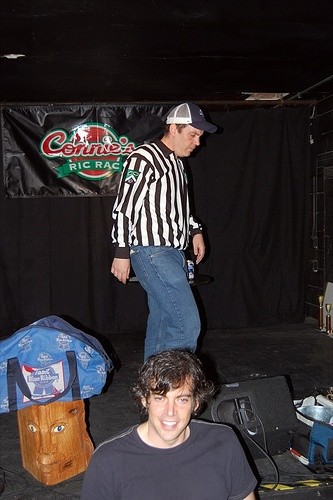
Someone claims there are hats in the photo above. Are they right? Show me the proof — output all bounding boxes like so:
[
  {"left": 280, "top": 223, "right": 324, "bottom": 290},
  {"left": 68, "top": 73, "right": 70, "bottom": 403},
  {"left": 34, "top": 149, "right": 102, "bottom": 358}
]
[{"left": 166, "top": 102, "right": 217, "bottom": 134}]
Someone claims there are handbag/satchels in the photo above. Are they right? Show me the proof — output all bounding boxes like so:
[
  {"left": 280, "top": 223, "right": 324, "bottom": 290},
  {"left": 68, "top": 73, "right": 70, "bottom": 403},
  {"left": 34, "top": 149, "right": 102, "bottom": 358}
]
[{"left": 0, "top": 316, "right": 114, "bottom": 414}]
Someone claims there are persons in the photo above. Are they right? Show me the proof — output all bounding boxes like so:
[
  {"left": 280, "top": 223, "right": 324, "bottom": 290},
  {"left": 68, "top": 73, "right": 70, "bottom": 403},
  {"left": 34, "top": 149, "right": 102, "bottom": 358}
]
[
  {"left": 110, "top": 102, "right": 217, "bottom": 363},
  {"left": 81, "top": 350, "right": 258, "bottom": 500}
]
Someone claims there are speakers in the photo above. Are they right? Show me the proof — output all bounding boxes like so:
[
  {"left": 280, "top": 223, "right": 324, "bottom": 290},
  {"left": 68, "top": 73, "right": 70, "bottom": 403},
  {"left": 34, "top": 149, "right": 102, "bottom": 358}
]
[{"left": 198, "top": 375, "right": 299, "bottom": 459}]
